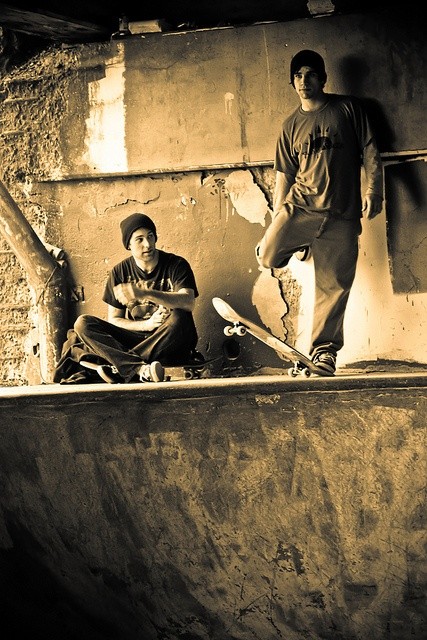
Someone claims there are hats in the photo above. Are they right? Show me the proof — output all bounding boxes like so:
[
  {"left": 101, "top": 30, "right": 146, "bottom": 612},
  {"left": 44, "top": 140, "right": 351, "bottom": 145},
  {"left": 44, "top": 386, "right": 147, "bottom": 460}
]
[
  {"left": 119, "top": 213, "right": 156, "bottom": 250},
  {"left": 289, "top": 50, "right": 326, "bottom": 88}
]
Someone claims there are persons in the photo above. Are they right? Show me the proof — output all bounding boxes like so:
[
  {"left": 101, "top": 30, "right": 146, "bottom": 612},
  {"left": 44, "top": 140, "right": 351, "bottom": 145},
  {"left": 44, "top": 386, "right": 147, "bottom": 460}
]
[
  {"left": 251, "top": 45, "right": 385, "bottom": 378},
  {"left": 72, "top": 211, "right": 200, "bottom": 386}
]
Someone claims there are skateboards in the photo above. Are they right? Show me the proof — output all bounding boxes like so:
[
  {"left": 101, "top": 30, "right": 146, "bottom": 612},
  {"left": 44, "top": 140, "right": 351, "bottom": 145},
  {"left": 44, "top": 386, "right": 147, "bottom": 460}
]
[
  {"left": 211, "top": 296, "right": 336, "bottom": 378},
  {"left": 161, "top": 355, "right": 224, "bottom": 379}
]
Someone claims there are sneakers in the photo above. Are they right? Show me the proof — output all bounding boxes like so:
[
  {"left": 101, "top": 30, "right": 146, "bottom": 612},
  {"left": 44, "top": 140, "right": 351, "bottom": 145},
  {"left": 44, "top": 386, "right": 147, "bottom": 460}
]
[
  {"left": 311, "top": 352, "right": 336, "bottom": 374},
  {"left": 294, "top": 245, "right": 311, "bottom": 262},
  {"left": 96, "top": 364, "right": 125, "bottom": 383},
  {"left": 139, "top": 360, "right": 162, "bottom": 382}
]
